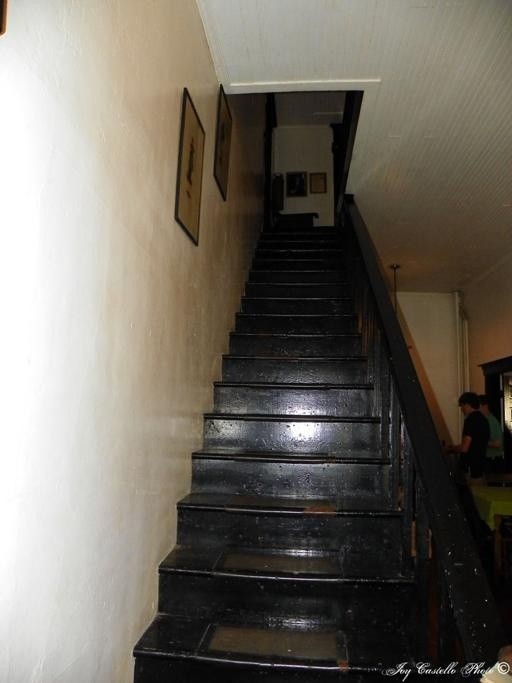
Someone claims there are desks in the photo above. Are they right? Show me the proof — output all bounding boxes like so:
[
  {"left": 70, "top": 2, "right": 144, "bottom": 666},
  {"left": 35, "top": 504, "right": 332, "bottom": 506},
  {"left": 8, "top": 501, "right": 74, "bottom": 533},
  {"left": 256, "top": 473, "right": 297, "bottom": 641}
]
[
  {"left": 472, "top": 484, "right": 511, "bottom": 573},
  {"left": 276, "top": 213, "right": 319, "bottom": 227}
]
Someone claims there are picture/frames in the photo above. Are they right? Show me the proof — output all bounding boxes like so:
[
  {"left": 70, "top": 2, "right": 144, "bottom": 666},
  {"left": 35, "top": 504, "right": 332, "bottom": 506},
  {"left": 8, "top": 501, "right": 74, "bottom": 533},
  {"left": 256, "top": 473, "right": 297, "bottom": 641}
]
[
  {"left": 309, "top": 172, "right": 326, "bottom": 193},
  {"left": 174, "top": 84, "right": 239, "bottom": 248}
]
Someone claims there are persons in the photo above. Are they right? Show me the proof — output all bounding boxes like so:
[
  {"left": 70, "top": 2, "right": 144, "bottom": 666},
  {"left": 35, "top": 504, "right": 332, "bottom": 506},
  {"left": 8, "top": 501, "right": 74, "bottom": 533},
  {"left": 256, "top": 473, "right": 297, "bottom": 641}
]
[
  {"left": 478, "top": 394, "right": 504, "bottom": 488},
  {"left": 444, "top": 391, "right": 490, "bottom": 540}
]
